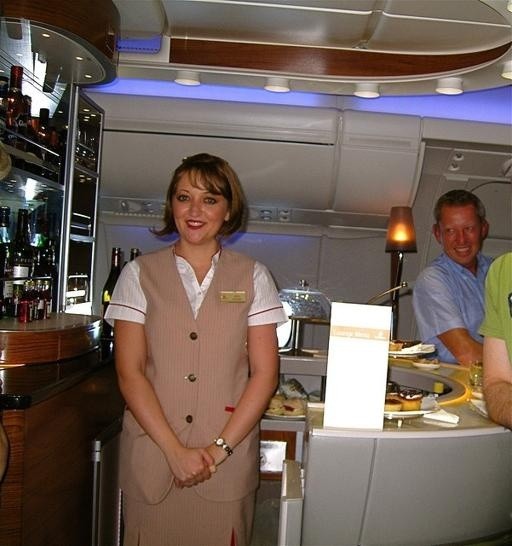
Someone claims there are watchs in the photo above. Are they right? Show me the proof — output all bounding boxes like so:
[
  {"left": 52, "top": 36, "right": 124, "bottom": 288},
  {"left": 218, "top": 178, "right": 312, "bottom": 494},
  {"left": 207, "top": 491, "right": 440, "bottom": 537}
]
[{"left": 212, "top": 437, "right": 233, "bottom": 456}]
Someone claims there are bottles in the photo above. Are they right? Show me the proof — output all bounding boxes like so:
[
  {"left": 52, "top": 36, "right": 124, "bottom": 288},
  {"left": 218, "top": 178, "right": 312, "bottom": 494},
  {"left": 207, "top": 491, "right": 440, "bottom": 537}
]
[
  {"left": 101, "top": 248, "right": 122, "bottom": 339},
  {"left": 1, "top": 202, "right": 56, "bottom": 322},
  {"left": 130, "top": 246, "right": 140, "bottom": 261},
  {"left": 0, "top": 63, "right": 58, "bottom": 159}
]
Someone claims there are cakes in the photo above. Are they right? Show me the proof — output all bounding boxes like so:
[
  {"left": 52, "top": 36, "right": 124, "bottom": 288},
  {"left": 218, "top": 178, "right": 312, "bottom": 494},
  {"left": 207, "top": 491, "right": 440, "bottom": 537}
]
[
  {"left": 389, "top": 339, "right": 422, "bottom": 352},
  {"left": 385, "top": 399, "right": 402, "bottom": 411},
  {"left": 387, "top": 392, "right": 423, "bottom": 410},
  {"left": 265, "top": 395, "right": 285, "bottom": 415},
  {"left": 283, "top": 398, "right": 306, "bottom": 416}
]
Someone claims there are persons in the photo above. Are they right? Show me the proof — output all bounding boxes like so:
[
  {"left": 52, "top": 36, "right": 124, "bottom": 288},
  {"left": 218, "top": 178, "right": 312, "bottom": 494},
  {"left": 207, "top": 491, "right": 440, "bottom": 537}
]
[
  {"left": 102, "top": 152, "right": 287, "bottom": 546},
  {"left": 479, "top": 250, "right": 512, "bottom": 430},
  {"left": 411, "top": 189, "right": 490, "bottom": 367}
]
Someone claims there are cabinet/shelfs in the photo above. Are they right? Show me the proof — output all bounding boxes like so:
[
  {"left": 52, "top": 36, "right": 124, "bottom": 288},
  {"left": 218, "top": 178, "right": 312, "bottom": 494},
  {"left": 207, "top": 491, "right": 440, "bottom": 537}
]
[{"left": 64, "top": 84, "right": 105, "bottom": 313}]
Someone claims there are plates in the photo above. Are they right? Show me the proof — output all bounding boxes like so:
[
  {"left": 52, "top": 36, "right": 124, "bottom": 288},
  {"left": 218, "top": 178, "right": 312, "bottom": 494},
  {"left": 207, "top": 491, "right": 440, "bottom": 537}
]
[
  {"left": 264, "top": 411, "right": 308, "bottom": 422},
  {"left": 385, "top": 410, "right": 432, "bottom": 421},
  {"left": 390, "top": 347, "right": 441, "bottom": 370}
]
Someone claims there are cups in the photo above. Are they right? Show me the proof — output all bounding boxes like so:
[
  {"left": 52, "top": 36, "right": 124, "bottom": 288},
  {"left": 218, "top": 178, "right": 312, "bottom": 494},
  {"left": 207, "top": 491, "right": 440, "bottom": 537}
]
[{"left": 469, "top": 360, "right": 482, "bottom": 389}]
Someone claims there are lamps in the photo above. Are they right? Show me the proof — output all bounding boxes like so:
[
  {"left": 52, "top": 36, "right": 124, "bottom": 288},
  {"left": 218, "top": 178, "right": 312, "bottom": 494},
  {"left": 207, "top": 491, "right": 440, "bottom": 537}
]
[
  {"left": 263, "top": 77, "right": 289, "bottom": 92},
  {"left": 501, "top": 61, "right": 512, "bottom": 79},
  {"left": 174, "top": 70, "right": 201, "bottom": 86},
  {"left": 507, "top": 0, "right": 512, "bottom": 12},
  {"left": 381, "top": 203, "right": 421, "bottom": 342},
  {"left": 353, "top": 84, "right": 380, "bottom": 99},
  {"left": 436, "top": 78, "right": 463, "bottom": 95}
]
